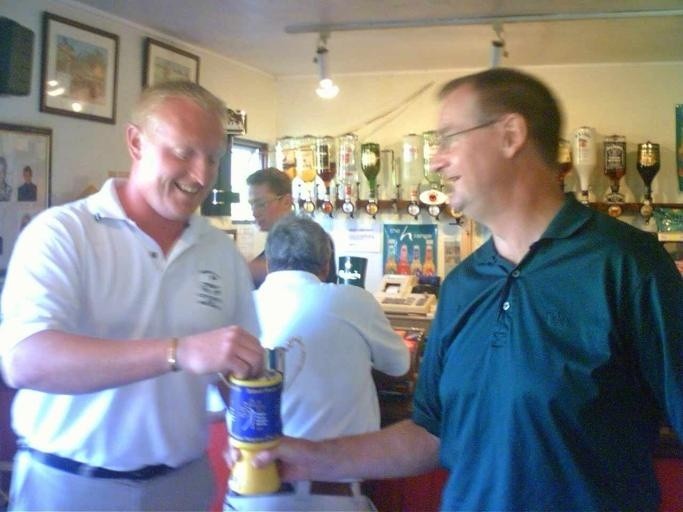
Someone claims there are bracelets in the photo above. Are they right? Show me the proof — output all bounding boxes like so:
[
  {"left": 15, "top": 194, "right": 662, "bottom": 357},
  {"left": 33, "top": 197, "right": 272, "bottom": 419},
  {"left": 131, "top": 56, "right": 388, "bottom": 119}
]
[{"left": 165, "top": 337, "right": 179, "bottom": 373}]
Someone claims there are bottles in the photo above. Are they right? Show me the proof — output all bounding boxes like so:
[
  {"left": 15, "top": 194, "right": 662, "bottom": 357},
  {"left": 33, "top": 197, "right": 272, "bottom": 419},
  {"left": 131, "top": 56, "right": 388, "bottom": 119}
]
[
  {"left": 604, "top": 137, "right": 627, "bottom": 218},
  {"left": 361, "top": 142, "right": 380, "bottom": 219},
  {"left": 277, "top": 138, "right": 296, "bottom": 214},
  {"left": 317, "top": 137, "right": 335, "bottom": 219},
  {"left": 557, "top": 138, "right": 571, "bottom": 191},
  {"left": 337, "top": 134, "right": 359, "bottom": 219},
  {"left": 401, "top": 134, "right": 422, "bottom": 219},
  {"left": 573, "top": 128, "right": 594, "bottom": 208},
  {"left": 422, "top": 239, "right": 434, "bottom": 276},
  {"left": 411, "top": 246, "right": 422, "bottom": 273},
  {"left": 399, "top": 246, "right": 411, "bottom": 273},
  {"left": 295, "top": 135, "right": 316, "bottom": 216},
  {"left": 423, "top": 131, "right": 442, "bottom": 220},
  {"left": 638, "top": 142, "right": 661, "bottom": 224},
  {"left": 386, "top": 238, "right": 397, "bottom": 274}
]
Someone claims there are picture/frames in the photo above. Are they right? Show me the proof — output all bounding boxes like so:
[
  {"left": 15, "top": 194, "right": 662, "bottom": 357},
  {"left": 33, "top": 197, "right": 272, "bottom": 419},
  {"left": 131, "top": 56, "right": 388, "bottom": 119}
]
[
  {"left": 39, "top": 11, "right": 120, "bottom": 125},
  {"left": 0, "top": 122, "right": 54, "bottom": 277},
  {"left": 142, "top": 36, "right": 201, "bottom": 88}
]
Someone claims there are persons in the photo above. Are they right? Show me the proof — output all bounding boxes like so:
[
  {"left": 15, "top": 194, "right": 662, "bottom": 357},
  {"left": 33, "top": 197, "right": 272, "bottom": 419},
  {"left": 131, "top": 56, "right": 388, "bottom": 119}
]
[
  {"left": 0, "top": 80, "right": 265, "bottom": 512},
  {"left": 223, "top": 217, "right": 409, "bottom": 512},
  {"left": 222, "top": 68, "right": 683, "bottom": 512},
  {"left": 245, "top": 167, "right": 337, "bottom": 291},
  {"left": 1, "top": 155, "right": 12, "bottom": 202},
  {"left": 17, "top": 165, "right": 37, "bottom": 201}
]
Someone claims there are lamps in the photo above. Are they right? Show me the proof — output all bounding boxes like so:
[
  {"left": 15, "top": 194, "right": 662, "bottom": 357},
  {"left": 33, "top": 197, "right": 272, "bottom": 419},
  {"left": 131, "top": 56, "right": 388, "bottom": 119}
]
[{"left": 314, "top": 47, "right": 339, "bottom": 101}]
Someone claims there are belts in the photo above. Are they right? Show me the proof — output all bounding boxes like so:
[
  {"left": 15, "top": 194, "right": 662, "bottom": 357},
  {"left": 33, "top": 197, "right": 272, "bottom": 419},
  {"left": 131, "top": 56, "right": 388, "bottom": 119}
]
[
  {"left": 29, "top": 448, "right": 170, "bottom": 481},
  {"left": 227, "top": 479, "right": 370, "bottom": 497}
]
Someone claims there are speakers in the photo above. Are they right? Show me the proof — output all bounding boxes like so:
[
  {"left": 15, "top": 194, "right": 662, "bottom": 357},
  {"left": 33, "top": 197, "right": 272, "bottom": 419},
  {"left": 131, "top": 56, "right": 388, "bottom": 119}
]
[{"left": 0, "top": 16, "right": 36, "bottom": 98}]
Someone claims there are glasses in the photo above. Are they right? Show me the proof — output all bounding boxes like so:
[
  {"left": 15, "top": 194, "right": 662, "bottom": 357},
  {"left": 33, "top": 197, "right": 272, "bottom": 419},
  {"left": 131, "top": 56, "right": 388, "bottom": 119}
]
[
  {"left": 431, "top": 120, "right": 494, "bottom": 146},
  {"left": 246, "top": 195, "right": 282, "bottom": 209}
]
